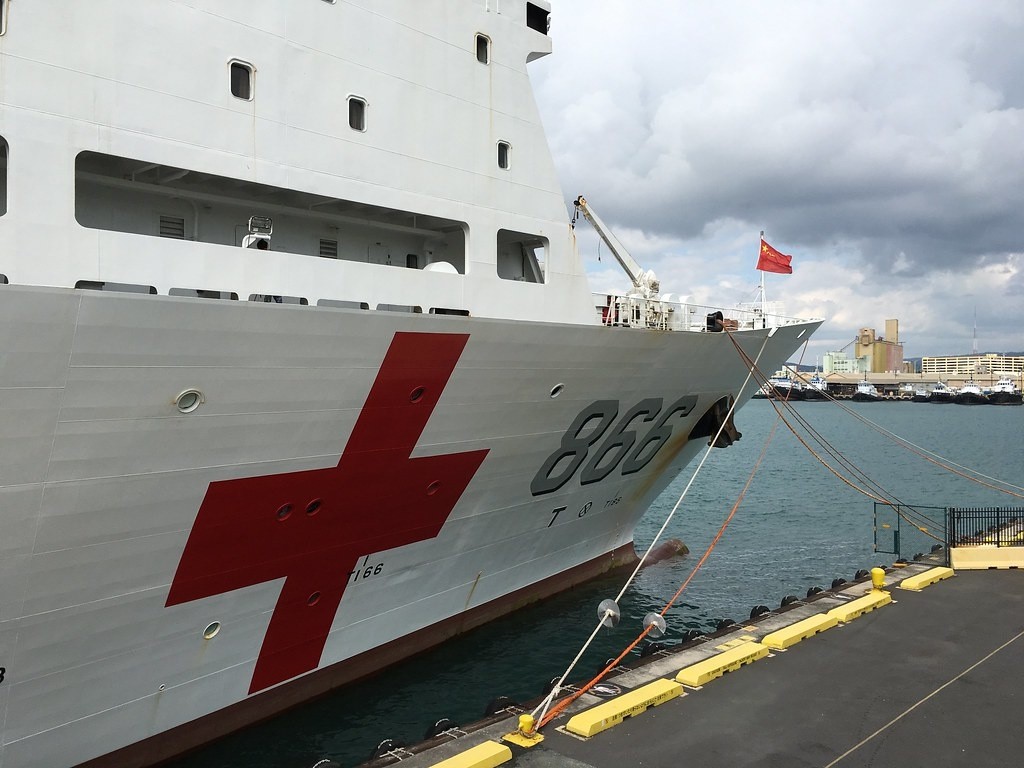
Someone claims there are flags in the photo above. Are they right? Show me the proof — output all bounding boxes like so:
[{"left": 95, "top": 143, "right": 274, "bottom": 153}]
[{"left": 756, "top": 239, "right": 793, "bottom": 274}]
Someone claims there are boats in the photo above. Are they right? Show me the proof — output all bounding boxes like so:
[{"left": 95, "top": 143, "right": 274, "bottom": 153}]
[
  {"left": 752, "top": 355, "right": 835, "bottom": 401},
  {"left": 914, "top": 373, "right": 1024, "bottom": 405},
  {"left": 851, "top": 370, "right": 883, "bottom": 401}
]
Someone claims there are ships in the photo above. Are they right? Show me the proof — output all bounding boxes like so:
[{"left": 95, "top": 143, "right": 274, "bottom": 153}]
[{"left": 3, "top": 0, "right": 828, "bottom": 768}]
